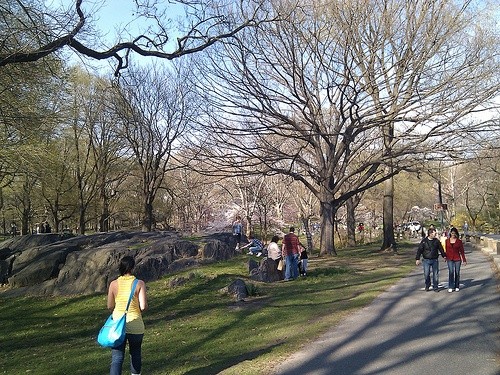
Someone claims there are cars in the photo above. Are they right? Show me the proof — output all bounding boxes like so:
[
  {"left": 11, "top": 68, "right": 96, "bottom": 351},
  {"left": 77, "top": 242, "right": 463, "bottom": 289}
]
[
  {"left": 409, "top": 221, "right": 421, "bottom": 232},
  {"left": 311, "top": 224, "right": 321, "bottom": 229}
]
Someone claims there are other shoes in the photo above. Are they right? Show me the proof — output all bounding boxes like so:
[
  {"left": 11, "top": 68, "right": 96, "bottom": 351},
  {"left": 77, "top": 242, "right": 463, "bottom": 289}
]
[
  {"left": 455, "top": 288, "right": 459, "bottom": 292},
  {"left": 246, "top": 252, "right": 253, "bottom": 255},
  {"left": 435, "top": 289, "right": 439, "bottom": 292},
  {"left": 256, "top": 253, "right": 262, "bottom": 257},
  {"left": 425, "top": 287, "right": 429, "bottom": 291},
  {"left": 448, "top": 288, "right": 452, "bottom": 292}
]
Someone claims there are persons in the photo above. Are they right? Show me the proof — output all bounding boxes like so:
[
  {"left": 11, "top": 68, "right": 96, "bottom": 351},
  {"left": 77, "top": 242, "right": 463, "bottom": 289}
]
[
  {"left": 107, "top": 255, "right": 148, "bottom": 375},
  {"left": 415, "top": 228, "right": 447, "bottom": 292},
  {"left": 8, "top": 221, "right": 52, "bottom": 237},
  {"left": 232, "top": 215, "right": 308, "bottom": 281},
  {"left": 425, "top": 223, "right": 448, "bottom": 254},
  {"left": 445, "top": 227, "right": 467, "bottom": 293}
]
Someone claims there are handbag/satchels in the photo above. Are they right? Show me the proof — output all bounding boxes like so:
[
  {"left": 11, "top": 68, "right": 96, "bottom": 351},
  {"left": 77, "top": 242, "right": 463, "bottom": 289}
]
[
  {"left": 97, "top": 313, "right": 128, "bottom": 348},
  {"left": 300, "top": 251, "right": 308, "bottom": 259},
  {"left": 277, "top": 256, "right": 285, "bottom": 270}
]
